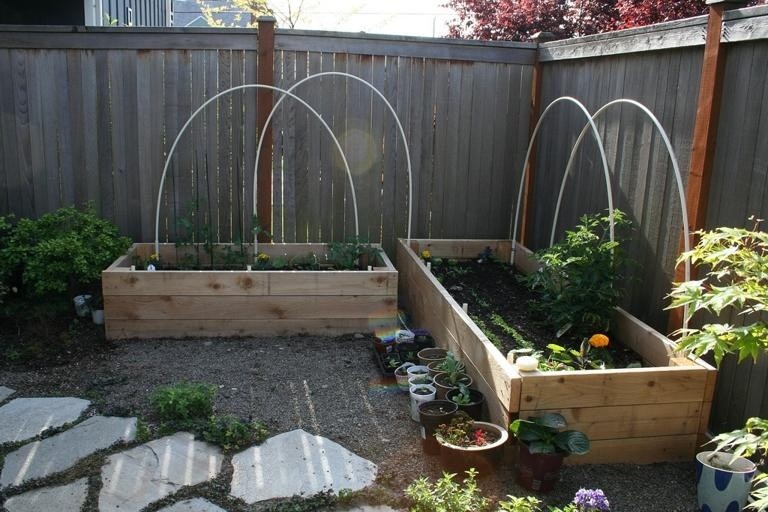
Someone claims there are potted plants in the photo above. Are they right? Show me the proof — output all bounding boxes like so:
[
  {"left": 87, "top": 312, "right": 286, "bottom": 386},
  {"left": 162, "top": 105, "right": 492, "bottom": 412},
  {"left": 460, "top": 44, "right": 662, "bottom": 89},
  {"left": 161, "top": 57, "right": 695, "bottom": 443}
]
[
  {"left": 433, "top": 351, "right": 473, "bottom": 400},
  {"left": 509, "top": 413, "right": 591, "bottom": 492},
  {"left": 445, "top": 383, "right": 486, "bottom": 421},
  {"left": 427, "top": 358, "right": 466, "bottom": 377},
  {"left": 83, "top": 290, "right": 105, "bottom": 324},
  {"left": 433, "top": 410, "right": 509, "bottom": 475},
  {"left": 383, "top": 361, "right": 402, "bottom": 372}
]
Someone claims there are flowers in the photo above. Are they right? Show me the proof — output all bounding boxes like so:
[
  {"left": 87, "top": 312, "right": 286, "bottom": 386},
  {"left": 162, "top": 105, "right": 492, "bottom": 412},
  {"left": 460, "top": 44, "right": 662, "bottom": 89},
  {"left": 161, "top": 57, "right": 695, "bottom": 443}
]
[
  {"left": 395, "top": 329, "right": 415, "bottom": 344},
  {"left": 409, "top": 327, "right": 432, "bottom": 341}
]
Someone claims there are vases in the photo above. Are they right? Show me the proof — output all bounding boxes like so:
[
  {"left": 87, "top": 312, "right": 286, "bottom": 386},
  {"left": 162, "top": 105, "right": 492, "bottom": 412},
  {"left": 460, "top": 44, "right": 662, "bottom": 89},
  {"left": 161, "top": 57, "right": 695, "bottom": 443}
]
[
  {"left": 409, "top": 384, "right": 436, "bottom": 423},
  {"left": 397, "top": 342, "right": 417, "bottom": 364},
  {"left": 695, "top": 451, "right": 758, "bottom": 512},
  {"left": 417, "top": 348, "right": 450, "bottom": 366},
  {"left": 417, "top": 399, "right": 459, "bottom": 457},
  {"left": 407, "top": 365, "right": 427, "bottom": 378},
  {"left": 380, "top": 353, "right": 398, "bottom": 361},
  {"left": 394, "top": 361, "right": 416, "bottom": 392},
  {"left": 414, "top": 335, "right": 436, "bottom": 352},
  {"left": 407, "top": 374, "right": 435, "bottom": 390},
  {"left": 73, "top": 294, "right": 92, "bottom": 317},
  {"left": 373, "top": 338, "right": 396, "bottom": 355}
]
